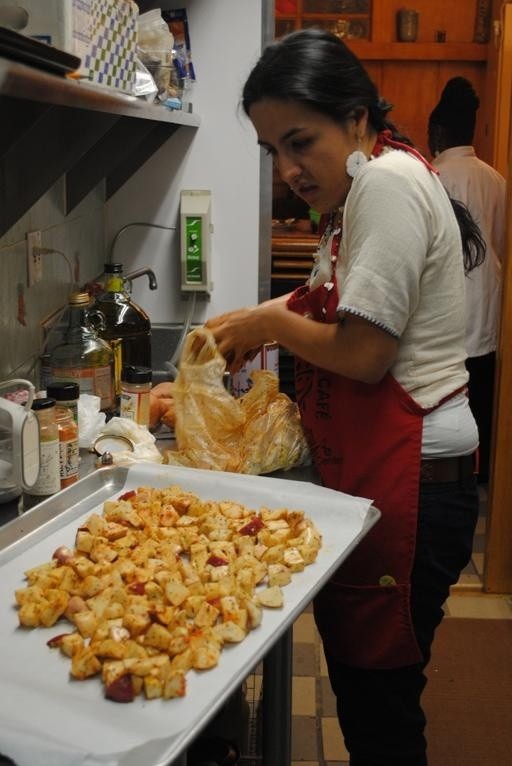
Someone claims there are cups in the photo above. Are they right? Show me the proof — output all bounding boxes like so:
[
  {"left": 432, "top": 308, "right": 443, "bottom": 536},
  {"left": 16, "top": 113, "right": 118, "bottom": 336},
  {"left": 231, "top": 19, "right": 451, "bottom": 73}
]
[
  {"left": 436, "top": 29, "right": 447, "bottom": 42},
  {"left": 395, "top": 8, "right": 419, "bottom": 42},
  {"left": 144, "top": 62, "right": 178, "bottom": 103}
]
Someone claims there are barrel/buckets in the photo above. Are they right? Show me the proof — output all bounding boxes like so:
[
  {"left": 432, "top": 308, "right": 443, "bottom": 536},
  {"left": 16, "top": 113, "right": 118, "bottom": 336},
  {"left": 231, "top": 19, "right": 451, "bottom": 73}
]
[
  {"left": 43, "top": 292, "right": 117, "bottom": 420},
  {"left": 92, "top": 262, "right": 152, "bottom": 432}
]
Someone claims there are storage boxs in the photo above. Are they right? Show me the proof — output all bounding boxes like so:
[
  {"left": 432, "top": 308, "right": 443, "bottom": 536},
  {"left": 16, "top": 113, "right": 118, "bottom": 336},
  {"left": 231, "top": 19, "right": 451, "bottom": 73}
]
[{"left": 0, "top": 1, "right": 139, "bottom": 95}]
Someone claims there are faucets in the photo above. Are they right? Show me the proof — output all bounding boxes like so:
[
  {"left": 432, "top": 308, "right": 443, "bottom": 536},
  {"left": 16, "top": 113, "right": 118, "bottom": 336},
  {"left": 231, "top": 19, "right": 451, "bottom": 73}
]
[{"left": 122, "top": 264, "right": 159, "bottom": 292}]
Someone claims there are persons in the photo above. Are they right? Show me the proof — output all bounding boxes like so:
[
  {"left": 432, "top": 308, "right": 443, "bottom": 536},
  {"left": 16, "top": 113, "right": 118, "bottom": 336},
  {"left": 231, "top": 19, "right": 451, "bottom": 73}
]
[
  {"left": 207, "top": 30, "right": 489, "bottom": 766},
  {"left": 425, "top": 102, "right": 509, "bottom": 484}
]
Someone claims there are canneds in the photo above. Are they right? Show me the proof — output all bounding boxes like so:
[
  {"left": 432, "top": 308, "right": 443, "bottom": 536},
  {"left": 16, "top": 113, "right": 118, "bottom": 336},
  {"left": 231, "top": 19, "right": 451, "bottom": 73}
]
[
  {"left": 228, "top": 340, "right": 280, "bottom": 400},
  {"left": 397, "top": 8, "right": 418, "bottom": 42},
  {"left": 56, "top": 406, "right": 79, "bottom": 490}
]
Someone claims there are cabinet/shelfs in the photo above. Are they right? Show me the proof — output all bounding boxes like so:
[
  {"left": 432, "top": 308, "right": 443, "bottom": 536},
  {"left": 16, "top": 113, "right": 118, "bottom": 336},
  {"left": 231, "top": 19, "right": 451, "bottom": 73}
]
[{"left": 274, "top": 1, "right": 391, "bottom": 62}]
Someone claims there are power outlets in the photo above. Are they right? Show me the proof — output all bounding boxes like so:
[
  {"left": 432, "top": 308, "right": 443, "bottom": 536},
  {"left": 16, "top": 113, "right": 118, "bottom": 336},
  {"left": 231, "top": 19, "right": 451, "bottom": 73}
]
[{"left": 27, "top": 229, "right": 43, "bottom": 289}]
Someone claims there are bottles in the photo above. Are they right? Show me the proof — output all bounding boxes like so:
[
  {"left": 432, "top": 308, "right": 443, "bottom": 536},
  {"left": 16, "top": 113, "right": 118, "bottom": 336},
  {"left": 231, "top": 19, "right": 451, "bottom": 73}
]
[
  {"left": 119, "top": 364, "right": 153, "bottom": 432},
  {"left": 21, "top": 381, "right": 81, "bottom": 498}
]
